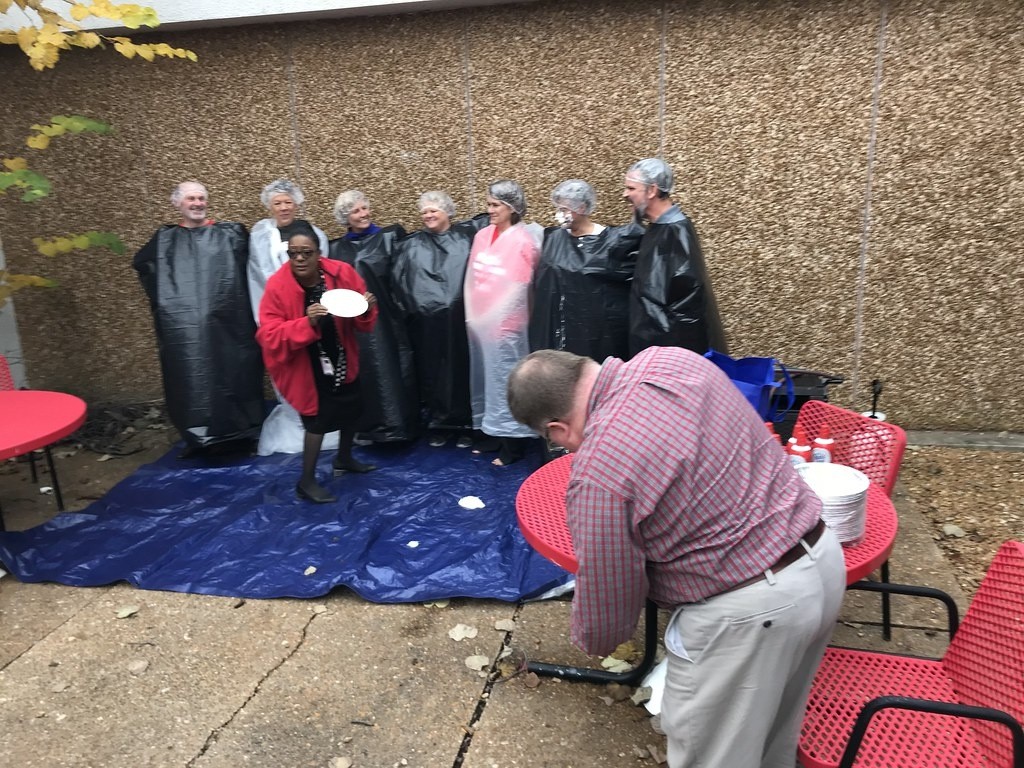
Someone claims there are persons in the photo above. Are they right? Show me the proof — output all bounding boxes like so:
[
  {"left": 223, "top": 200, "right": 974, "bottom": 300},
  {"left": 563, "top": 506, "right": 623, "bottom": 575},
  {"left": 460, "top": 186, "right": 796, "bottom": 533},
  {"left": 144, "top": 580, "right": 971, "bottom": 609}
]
[
  {"left": 131, "top": 180, "right": 251, "bottom": 448},
  {"left": 622, "top": 157, "right": 727, "bottom": 366},
  {"left": 392, "top": 190, "right": 471, "bottom": 448},
  {"left": 464, "top": 181, "right": 540, "bottom": 468},
  {"left": 326, "top": 189, "right": 402, "bottom": 449},
  {"left": 255, "top": 226, "right": 377, "bottom": 505},
  {"left": 527, "top": 180, "right": 625, "bottom": 365},
  {"left": 508, "top": 344, "right": 846, "bottom": 768},
  {"left": 248, "top": 176, "right": 333, "bottom": 453}
]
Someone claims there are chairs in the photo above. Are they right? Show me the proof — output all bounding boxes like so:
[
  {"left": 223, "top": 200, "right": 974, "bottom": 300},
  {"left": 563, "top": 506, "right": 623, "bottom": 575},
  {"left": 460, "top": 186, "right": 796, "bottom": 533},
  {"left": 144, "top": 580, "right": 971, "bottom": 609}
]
[
  {"left": 0, "top": 355, "right": 15, "bottom": 391},
  {"left": 797, "top": 538, "right": 1024, "bottom": 768},
  {"left": 793, "top": 400, "right": 906, "bottom": 498}
]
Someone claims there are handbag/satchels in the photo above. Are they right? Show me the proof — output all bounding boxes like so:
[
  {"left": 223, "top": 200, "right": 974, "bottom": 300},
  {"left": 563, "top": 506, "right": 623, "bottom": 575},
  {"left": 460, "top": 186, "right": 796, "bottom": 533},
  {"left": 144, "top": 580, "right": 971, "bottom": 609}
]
[{"left": 703, "top": 350, "right": 795, "bottom": 422}]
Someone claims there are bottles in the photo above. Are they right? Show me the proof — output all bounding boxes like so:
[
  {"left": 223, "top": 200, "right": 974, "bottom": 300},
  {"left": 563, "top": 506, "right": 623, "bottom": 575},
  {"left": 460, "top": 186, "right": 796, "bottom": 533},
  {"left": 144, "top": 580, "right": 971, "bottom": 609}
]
[
  {"left": 812, "top": 424, "right": 835, "bottom": 463},
  {"left": 786, "top": 424, "right": 811, "bottom": 464},
  {"left": 764, "top": 420, "right": 781, "bottom": 446}
]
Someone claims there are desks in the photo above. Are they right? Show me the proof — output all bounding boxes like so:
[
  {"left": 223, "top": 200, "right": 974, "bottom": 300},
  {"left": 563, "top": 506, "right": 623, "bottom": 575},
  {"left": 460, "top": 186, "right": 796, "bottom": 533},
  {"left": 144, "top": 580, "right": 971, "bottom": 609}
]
[
  {"left": 515, "top": 451, "right": 901, "bottom": 641},
  {"left": 0, "top": 389, "right": 87, "bottom": 532}
]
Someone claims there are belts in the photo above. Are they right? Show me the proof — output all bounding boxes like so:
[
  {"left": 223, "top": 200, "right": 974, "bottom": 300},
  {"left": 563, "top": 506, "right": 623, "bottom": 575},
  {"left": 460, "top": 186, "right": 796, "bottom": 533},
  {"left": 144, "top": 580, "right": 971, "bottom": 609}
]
[{"left": 706, "top": 518, "right": 826, "bottom": 596}]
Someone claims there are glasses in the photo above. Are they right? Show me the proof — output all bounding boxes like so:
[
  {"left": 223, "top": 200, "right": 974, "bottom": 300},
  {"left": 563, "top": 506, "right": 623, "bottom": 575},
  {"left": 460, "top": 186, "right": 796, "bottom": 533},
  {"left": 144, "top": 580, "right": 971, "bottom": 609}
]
[{"left": 287, "top": 249, "right": 318, "bottom": 260}]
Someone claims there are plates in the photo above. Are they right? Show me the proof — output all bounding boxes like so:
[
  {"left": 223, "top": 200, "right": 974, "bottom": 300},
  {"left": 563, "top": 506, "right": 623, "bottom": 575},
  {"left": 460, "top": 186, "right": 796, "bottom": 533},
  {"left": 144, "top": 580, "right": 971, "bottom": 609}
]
[
  {"left": 318, "top": 288, "right": 370, "bottom": 318},
  {"left": 797, "top": 463, "right": 871, "bottom": 544}
]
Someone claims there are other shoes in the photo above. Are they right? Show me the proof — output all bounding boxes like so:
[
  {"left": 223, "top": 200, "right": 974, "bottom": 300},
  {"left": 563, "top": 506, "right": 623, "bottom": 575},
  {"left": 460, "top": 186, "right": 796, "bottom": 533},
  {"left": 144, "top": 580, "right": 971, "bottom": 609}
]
[
  {"left": 541, "top": 441, "right": 561, "bottom": 465},
  {"left": 175, "top": 445, "right": 198, "bottom": 460},
  {"left": 428, "top": 429, "right": 457, "bottom": 446},
  {"left": 332, "top": 455, "right": 377, "bottom": 474},
  {"left": 490, "top": 452, "right": 515, "bottom": 466},
  {"left": 248, "top": 439, "right": 260, "bottom": 457},
  {"left": 296, "top": 480, "right": 337, "bottom": 504},
  {"left": 455, "top": 430, "right": 481, "bottom": 448},
  {"left": 472, "top": 436, "right": 500, "bottom": 454}
]
